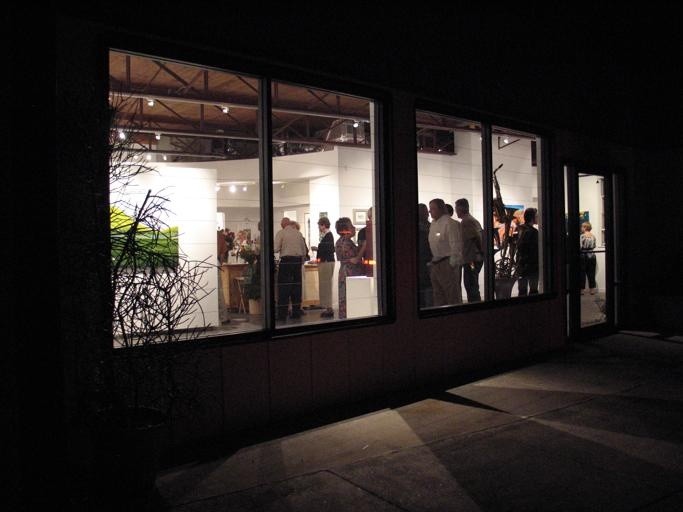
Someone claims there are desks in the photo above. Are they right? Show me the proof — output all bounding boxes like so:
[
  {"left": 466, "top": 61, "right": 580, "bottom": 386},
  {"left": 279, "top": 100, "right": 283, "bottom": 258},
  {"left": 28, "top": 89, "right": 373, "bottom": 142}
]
[{"left": 217, "top": 260, "right": 321, "bottom": 317}]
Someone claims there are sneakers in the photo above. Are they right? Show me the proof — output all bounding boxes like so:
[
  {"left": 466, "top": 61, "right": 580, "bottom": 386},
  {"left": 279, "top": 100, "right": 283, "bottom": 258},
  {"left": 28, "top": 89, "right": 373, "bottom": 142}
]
[
  {"left": 581, "top": 288, "right": 595, "bottom": 295},
  {"left": 320, "top": 311, "right": 334, "bottom": 317}
]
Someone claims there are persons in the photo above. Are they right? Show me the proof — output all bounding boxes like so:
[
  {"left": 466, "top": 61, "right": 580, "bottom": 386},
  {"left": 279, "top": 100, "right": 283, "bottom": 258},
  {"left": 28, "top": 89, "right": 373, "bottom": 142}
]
[{"left": 578, "top": 220, "right": 599, "bottom": 297}]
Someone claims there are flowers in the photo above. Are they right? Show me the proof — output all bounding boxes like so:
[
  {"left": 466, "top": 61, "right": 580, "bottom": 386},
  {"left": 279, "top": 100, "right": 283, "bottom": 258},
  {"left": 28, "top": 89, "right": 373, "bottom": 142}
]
[
  {"left": 237, "top": 240, "right": 259, "bottom": 258},
  {"left": 216, "top": 226, "right": 235, "bottom": 264}
]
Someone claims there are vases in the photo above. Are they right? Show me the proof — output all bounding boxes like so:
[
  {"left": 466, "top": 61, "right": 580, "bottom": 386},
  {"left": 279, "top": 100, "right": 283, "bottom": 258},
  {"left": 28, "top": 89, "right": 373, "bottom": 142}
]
[{"left": 241, "top": 255, "right": 256, "bottom": 285}]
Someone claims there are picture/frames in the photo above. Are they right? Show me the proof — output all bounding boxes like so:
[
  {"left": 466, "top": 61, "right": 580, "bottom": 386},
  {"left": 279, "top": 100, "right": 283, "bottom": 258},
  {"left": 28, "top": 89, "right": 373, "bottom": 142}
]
[{"left": 351, "top": 206, "right": 370, "bottom": 244}]
